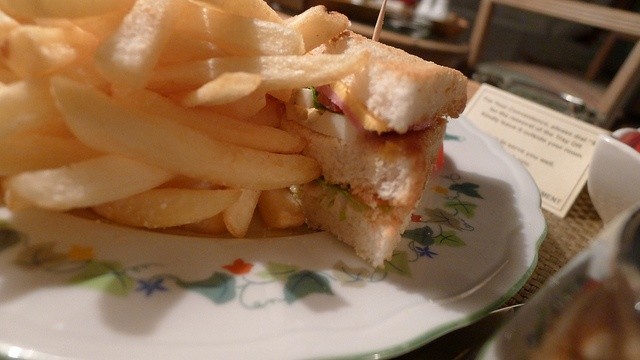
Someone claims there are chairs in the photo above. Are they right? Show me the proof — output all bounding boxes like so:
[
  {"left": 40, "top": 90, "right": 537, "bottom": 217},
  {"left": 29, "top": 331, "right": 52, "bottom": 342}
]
[{"left": 463, "top": 0, "right": 640, "bottom": 119}]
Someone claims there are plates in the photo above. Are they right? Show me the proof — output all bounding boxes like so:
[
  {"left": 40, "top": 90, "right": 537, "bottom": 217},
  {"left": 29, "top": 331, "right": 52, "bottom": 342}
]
[
  {"left": 476, "top": 202, "right": 640, "bottom": 360},
  {"left": 1, "top": 114, "right": 546, "bottom": 359}
]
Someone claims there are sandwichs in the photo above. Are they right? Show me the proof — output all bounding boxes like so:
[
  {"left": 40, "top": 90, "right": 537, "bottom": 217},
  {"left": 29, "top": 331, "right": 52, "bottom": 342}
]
[{"left": 292, "top": 26, "right": 468, "bottom": 268}]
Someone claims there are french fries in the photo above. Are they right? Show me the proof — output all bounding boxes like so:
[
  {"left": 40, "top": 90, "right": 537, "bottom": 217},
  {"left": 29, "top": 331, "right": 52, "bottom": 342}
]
[{"left": 1, "top": 0, "right": 369, "bottom": 246}]
